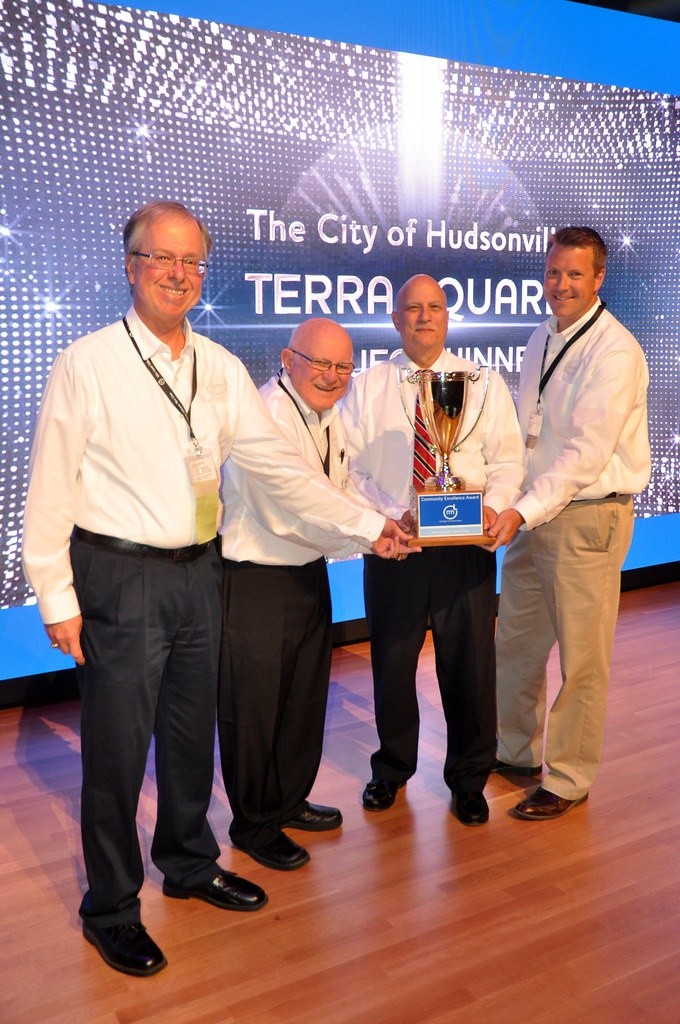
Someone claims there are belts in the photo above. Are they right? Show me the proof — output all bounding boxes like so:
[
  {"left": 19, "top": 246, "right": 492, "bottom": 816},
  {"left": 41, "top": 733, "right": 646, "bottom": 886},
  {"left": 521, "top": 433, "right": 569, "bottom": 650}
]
[
  {"left": 412, "top": 370, "right": 437, "bottom": 494},
  {"left": 606, "top": 492, "right": 623, "bottom": 499},
  {"left": 72, "top": 524, "right": 215, "bottom": 564}
]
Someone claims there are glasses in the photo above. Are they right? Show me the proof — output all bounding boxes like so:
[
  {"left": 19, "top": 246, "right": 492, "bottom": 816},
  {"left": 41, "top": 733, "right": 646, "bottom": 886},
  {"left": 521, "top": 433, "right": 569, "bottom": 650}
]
[
  {"left": 134, "top": 251, "right": 209, "bottom": 275},
  {"left": 292, "top": 351, "right": 355, "bottom": 375}
]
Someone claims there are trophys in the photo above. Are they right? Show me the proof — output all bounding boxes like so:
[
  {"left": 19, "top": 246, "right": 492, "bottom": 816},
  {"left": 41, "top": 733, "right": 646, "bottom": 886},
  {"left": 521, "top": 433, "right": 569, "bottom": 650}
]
[{"left": 397, "top": 365, "right": 484, "bottom": 492}]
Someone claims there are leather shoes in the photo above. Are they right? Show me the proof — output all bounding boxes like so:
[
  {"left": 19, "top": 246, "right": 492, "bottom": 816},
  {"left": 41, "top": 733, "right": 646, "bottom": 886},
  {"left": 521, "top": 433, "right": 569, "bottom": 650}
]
[
  {"left": 451, "top": 789, "right": 490, "bottom": 826},
  {"left": 249, "top": 831, "right": 310, "bottom": 871},
  {"left": 513, "top": 787, "right": 589, "bottom": 820},
  {"left": 162, "top": 869, "right": 267, "bottom": 912},
  {"left": 362, "top": 774, "right": 407, "bottom": 811},
  {"left": 82, "top": 918, "right": 167, "bottom": 976},
  {"left": 280, "top": 803, "right": 343, "bottom": 832},
  {"left": 491, "top": 757, "right": 542, "bottom": 776}
]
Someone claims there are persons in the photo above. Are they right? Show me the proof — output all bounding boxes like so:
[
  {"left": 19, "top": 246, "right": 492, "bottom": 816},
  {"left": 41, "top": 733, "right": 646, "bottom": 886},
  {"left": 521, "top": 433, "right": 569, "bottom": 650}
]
[
  {"left": 487, "top": 224, "right": 654, "bottom": 823},
  {"left": 20, "top": 197, "right": 422, "bottom": 976},
  {"left": 217, "top": 315, "right": 422, "bottom": 871},
  {"left": 341, "top": 273, "right": 530, "bottom": 825}
]
